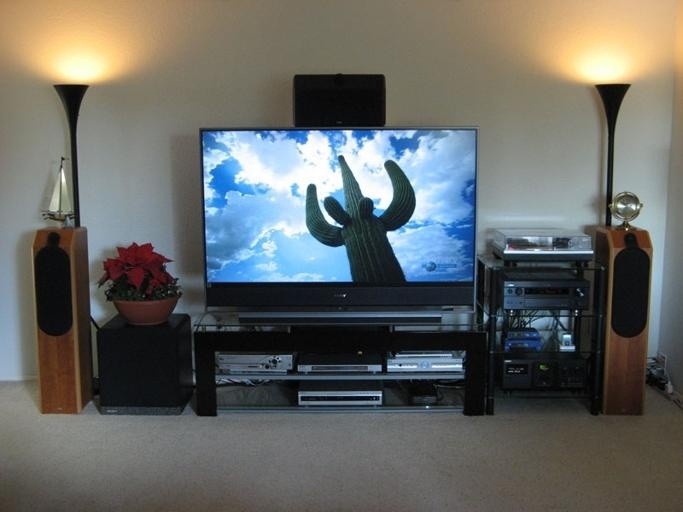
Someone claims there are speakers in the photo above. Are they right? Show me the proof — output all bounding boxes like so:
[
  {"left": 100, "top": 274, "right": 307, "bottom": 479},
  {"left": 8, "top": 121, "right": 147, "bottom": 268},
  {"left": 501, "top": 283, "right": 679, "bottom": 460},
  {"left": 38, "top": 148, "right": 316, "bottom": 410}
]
[
  {"left": 31, "top": 226, "right": 94, "bottom": 414},
  {"left": 584, "top": 225, "right": 653, "bottom": 416},
  {"left": 293, "top": 74, "right": 386, "bottom": 127}
]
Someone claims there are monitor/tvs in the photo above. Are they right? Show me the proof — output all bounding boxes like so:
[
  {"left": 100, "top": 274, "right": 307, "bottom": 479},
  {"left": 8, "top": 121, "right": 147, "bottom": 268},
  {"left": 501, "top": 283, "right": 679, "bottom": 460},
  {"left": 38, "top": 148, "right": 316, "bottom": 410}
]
[{"left": 199, "top": 126, "right": 478, "bottom": 322}]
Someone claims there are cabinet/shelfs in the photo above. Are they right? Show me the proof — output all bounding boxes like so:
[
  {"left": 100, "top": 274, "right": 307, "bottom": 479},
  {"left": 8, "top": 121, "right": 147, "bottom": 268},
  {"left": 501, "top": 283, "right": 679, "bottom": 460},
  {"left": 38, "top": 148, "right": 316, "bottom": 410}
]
[{"left": 478, "top": 252, "right": 605, "bottom": 416}]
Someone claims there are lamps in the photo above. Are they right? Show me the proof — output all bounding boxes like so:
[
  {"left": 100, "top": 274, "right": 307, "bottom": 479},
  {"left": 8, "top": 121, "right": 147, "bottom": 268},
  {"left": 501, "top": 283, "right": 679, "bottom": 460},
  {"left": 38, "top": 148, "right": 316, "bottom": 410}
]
[
  {"left": 54, "top": 84, "right": 90, "bottom": 228},
  {"left": 594, "top": 83, "right": 631, "bottom": 226}
]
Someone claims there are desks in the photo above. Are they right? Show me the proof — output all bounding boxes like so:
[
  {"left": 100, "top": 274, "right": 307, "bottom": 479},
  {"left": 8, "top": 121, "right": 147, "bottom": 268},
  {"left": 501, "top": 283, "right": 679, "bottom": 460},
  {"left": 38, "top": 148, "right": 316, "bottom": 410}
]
[{"left": 193, "top": 309, "right": 488, "bottom": 417}]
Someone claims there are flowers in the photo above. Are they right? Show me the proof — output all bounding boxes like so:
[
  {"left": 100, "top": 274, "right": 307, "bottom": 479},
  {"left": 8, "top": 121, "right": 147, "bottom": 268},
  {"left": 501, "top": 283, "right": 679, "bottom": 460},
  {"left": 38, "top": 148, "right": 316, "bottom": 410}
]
[{"left": 95, "top": 242, "right": 185, "bottom": 300}]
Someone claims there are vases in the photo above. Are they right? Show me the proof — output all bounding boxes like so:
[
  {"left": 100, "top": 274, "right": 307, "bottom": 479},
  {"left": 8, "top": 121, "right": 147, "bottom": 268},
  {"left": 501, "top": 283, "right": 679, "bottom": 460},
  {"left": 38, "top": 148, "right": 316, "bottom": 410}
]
[{"left": 111, "top": 299, "right": 179, "bottom": 325}]
